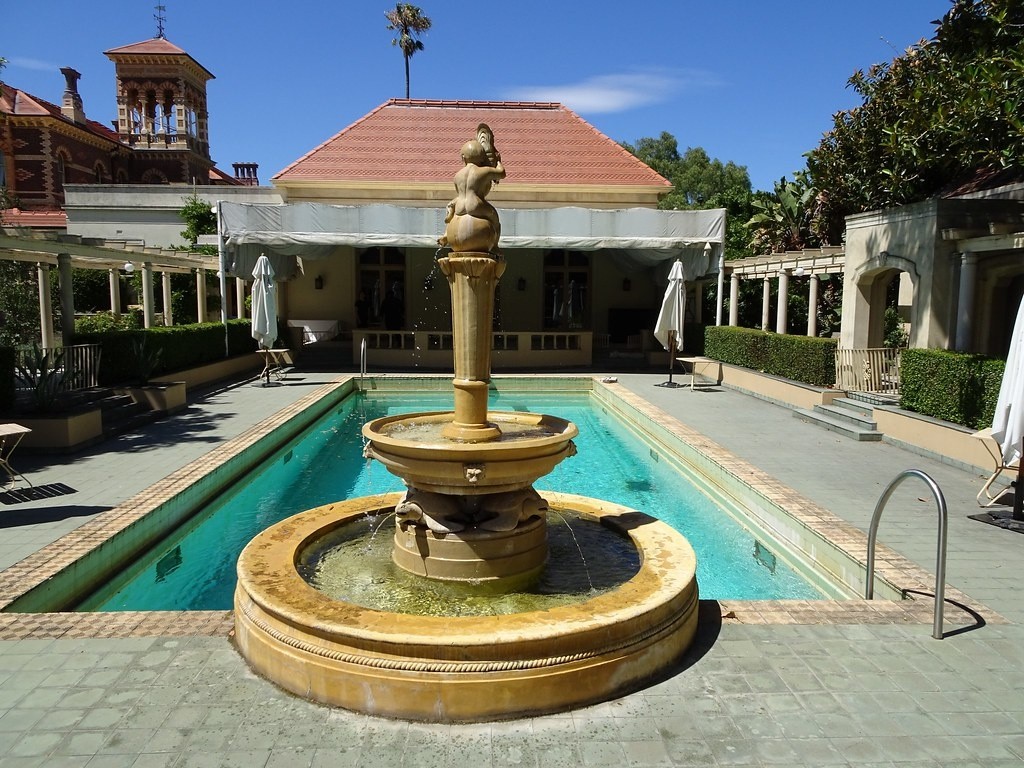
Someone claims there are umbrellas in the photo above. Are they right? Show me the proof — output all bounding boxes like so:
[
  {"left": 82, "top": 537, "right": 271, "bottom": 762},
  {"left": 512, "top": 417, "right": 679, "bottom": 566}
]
[
  {"left": 991, "top": 295, "right": 1024, "bottom": 520},
  {"left": 251, "top": 252, "right": 277, "bottom": 383},
  {"left": 653, "top": 258, "right": 684, "bottom": 383}
]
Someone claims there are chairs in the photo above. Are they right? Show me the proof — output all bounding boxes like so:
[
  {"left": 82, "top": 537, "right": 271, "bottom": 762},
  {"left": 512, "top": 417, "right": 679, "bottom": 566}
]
[{"left": 970, "top": 426, "right": 1019, "bottom": 507}]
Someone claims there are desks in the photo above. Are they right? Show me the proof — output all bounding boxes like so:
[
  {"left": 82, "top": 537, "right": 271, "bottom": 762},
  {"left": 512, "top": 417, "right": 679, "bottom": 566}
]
[
  {"left": 0, "top": 423, "right": 34, "bottom": 495},
  {"left": 255, "top": 349, "right": 289, "bottom": 387},
  {"left": 676, "top": 358, "right": 715, "bottom": 392}
]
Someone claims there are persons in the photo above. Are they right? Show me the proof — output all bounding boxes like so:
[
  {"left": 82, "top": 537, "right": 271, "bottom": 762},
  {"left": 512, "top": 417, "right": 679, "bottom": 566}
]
[
  {"left": 437, "top": 139, "right": 507, "bottom": 258},
  {"left": 353, "top": 291, "right": 404, "bottom": 348}
]
